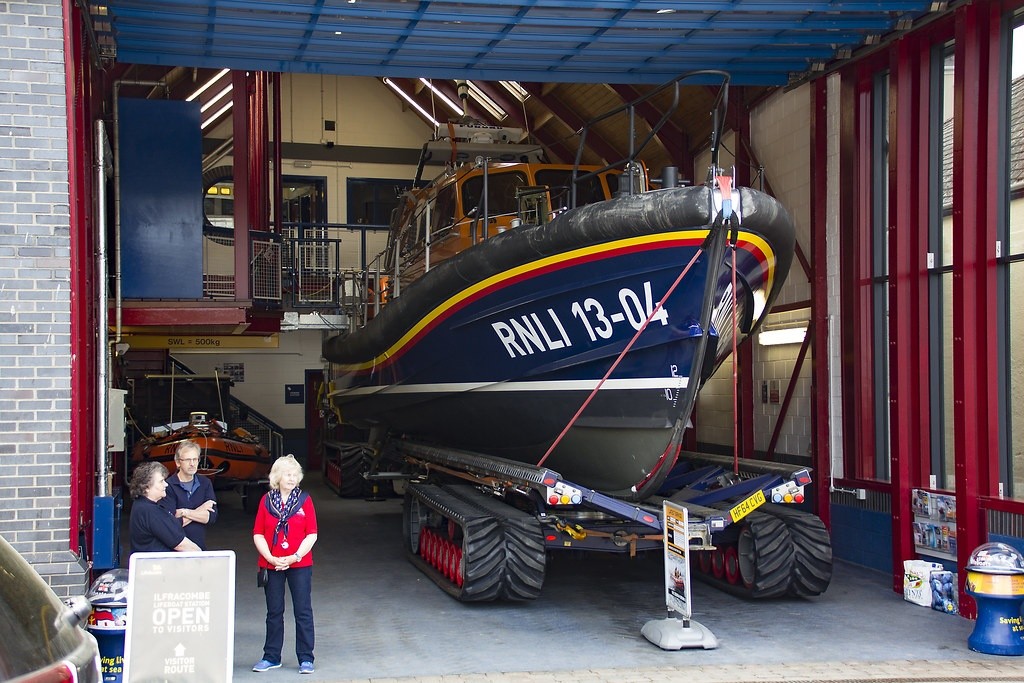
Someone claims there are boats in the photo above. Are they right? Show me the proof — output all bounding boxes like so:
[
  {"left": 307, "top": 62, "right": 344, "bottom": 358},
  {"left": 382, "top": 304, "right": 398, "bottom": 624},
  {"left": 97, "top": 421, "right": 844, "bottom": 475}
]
[
  {"left": 320, "top": 81, "right": 796, "bottom": 503},
  {"left": 131, "top": 410, "right": 273, "bottom": 482}
]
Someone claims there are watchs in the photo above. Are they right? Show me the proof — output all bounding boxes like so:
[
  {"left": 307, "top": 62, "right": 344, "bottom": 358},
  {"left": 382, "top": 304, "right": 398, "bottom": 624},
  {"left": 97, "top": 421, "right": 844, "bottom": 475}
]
[{"left": 294, "top": 553, "right": 302, "bottom": 563}]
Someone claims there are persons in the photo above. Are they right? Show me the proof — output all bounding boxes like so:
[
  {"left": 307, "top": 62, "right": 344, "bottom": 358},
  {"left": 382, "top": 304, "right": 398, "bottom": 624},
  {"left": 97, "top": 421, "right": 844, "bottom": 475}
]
[
  {"left": 130, "top": 462, "right": 202, "bottom": 553},
  {"left": 157, "top": 441, "right": 217, "bottom": 552},
  {"left": 252, "top": 454, "right": 318, "bottom": 674}
]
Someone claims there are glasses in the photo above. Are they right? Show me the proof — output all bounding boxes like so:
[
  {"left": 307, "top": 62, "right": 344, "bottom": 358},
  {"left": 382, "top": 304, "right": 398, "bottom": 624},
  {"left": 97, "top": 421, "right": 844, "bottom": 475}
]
[{"left": 177, "top": 458, "right": 201, "bottom": 463}]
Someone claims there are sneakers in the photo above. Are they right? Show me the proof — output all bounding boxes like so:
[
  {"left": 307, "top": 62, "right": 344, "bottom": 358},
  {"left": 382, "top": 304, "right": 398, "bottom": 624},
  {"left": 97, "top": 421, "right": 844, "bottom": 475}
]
[
  {"left": 298, "top": 660, "right": 314, "bottom": 673},
  {"left": 252, "top": 658, "right": 283, "bottom": 671}
]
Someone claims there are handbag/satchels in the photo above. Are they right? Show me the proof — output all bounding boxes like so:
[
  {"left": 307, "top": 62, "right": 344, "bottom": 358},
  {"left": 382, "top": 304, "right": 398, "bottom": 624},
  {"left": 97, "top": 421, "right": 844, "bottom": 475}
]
[
  {"left": 902, "top": 560, "right": 960, "bottom": 616},
  {"left": 257, "top": 568, "right": 268, "bottom": 588}
]
